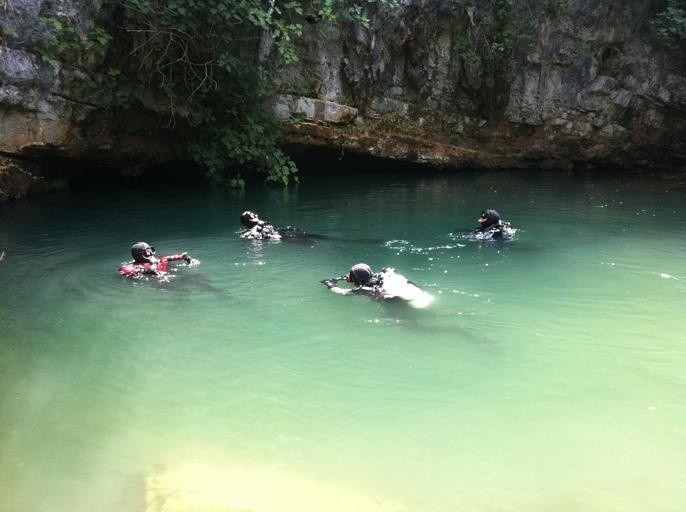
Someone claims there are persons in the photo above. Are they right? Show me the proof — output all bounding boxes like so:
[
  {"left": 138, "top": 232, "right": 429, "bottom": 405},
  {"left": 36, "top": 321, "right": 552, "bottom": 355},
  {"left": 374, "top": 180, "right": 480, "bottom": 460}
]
[
  {"left": 240, "top": 209, "right": 388, "bottom": 248},
  {"left": 317, "top": 264, "right": 436, "bottom": 312},
  {"left": 118, "top": 242, "right": 201, "bottom": 279},
  {"left": 468, "top": 210, "right": 514, "bottom": 245}
]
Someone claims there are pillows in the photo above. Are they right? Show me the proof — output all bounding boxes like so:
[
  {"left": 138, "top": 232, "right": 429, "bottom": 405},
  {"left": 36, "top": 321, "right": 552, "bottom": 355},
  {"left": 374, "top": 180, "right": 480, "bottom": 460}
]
[
  {"left": 351, "top": 263, "right": 373, "bottom": 285},
  {"left": 131, "top": 242, "right": 154, "bottom": 262},
  {"left": 482, "top": 209, "right": 500, "bottom": 227},
  {"left": 241, "top": 210, "right": 259, "bottom": 226}
]
